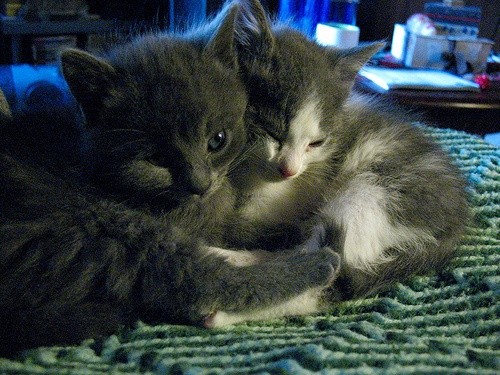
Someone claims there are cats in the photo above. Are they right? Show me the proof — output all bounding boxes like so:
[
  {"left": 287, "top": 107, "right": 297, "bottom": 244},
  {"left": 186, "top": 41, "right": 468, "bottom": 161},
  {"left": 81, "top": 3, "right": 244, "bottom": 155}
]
[{"left": 1, "top": 1, "right": 468, "bottom": 359}]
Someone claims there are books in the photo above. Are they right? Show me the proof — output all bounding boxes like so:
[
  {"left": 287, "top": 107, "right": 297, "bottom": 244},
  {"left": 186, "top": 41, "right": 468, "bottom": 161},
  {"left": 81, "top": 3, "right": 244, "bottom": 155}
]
[{"left": 358, "top": 65, "right": 484, "bottom": 93}]
